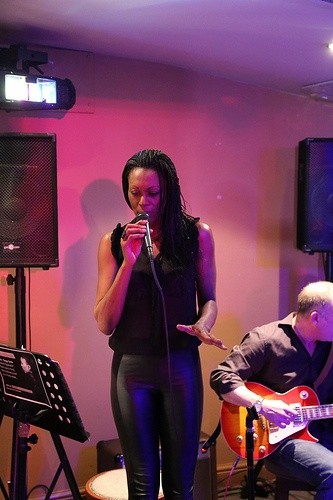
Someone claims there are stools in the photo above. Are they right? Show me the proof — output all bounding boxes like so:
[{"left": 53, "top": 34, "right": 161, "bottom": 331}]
[{"left": 85, "top": 469, "right": 165, "bottom": 500}]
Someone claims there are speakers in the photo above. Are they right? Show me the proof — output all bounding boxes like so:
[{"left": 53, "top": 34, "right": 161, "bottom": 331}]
[
  {"left": 0, "top": 133, "right": 60, "bottom": 271},
  {"left": 296, "top": 137, "right": 333, "bottom": 254},
  {"left": 96, "top": 429, "right": 218, "bottom": 500}
]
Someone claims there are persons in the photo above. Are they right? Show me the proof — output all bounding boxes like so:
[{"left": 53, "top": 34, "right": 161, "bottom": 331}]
[
  {"left": 210, "top": 281, "right": 333, "bottom": 500},
  {"left": 93, "top": 149, "right": 226, "bottom": 500}
]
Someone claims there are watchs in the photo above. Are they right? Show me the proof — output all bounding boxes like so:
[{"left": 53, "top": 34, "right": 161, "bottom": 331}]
[{"left": 253, "top": 397, "right": 265, "bottom": 414}]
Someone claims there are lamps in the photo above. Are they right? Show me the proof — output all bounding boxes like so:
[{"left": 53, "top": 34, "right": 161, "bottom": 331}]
[{"left": 0, "top": 42, "right": 77, "bottom": 110}]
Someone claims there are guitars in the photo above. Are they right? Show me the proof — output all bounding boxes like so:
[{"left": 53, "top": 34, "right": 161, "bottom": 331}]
[{"left": 218, "top": 381, "right": 333, "bottom": 460}]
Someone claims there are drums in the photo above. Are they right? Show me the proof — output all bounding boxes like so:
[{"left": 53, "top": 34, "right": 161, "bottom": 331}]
[{"left": 83, "top": 465, "right": 164, "bottom": 500}]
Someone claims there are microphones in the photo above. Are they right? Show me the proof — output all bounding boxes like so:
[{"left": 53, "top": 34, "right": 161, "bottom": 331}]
[{"left": 137, "top": 212, "right": 154, "bottom": 263}]
